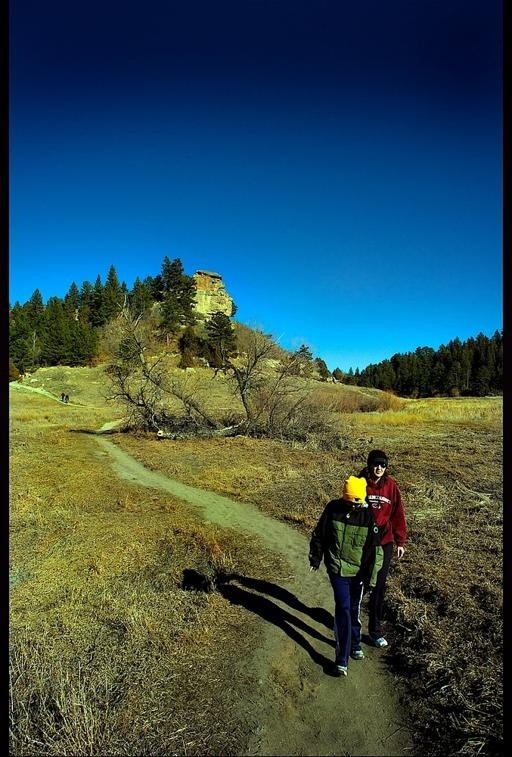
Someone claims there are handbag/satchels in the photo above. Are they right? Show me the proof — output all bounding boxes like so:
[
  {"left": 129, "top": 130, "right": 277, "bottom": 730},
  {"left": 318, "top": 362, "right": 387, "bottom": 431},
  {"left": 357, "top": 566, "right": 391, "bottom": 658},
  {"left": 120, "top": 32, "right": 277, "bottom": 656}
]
[{"left": 372, "top": 525, "right": 388, "bottom": 545}]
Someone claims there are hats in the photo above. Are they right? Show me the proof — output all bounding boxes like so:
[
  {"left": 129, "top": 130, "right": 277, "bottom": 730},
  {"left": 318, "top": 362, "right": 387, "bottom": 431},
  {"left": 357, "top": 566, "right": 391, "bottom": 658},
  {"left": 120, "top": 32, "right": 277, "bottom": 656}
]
[
  {"left": 343, "top": 476, "right": 368, "bottom": 503},
  {"left": 368, "top": 450, "right": 388, "bottom": 467}
]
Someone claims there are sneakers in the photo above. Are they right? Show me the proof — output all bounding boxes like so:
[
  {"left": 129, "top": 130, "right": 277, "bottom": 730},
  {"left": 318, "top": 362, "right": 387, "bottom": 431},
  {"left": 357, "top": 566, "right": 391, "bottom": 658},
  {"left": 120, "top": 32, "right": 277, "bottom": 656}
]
[
  {"left": 335, "top": 665, "right": 347, "bottom": 676},
  {"left": 351, "top": 651, "right": 365, "bottom": 659},
  {"left": 368, "top": 635, "right": 388, "bottom": 647}
]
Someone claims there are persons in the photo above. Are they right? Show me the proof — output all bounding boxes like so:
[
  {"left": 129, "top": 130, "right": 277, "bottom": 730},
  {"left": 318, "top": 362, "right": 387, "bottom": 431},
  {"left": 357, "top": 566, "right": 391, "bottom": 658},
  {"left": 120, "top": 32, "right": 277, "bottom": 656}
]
[
  {"left": 308, "top": 475, "right": 384, "bottom": 677},
  {"left": 60, "top": 391, "right": 64, "bottom": 402},
  {"left": 358, "top": 450, "right": 407, "bottom": 649},
  {"left": 65, "top": 395, "right": 68, "bottom": 402}
]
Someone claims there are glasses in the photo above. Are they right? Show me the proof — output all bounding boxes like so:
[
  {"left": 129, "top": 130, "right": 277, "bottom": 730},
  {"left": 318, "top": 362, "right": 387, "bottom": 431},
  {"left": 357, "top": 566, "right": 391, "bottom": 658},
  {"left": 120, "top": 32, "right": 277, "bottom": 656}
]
[{"left": 373, "top": 463, "right": 386, "bottom": 467}]
